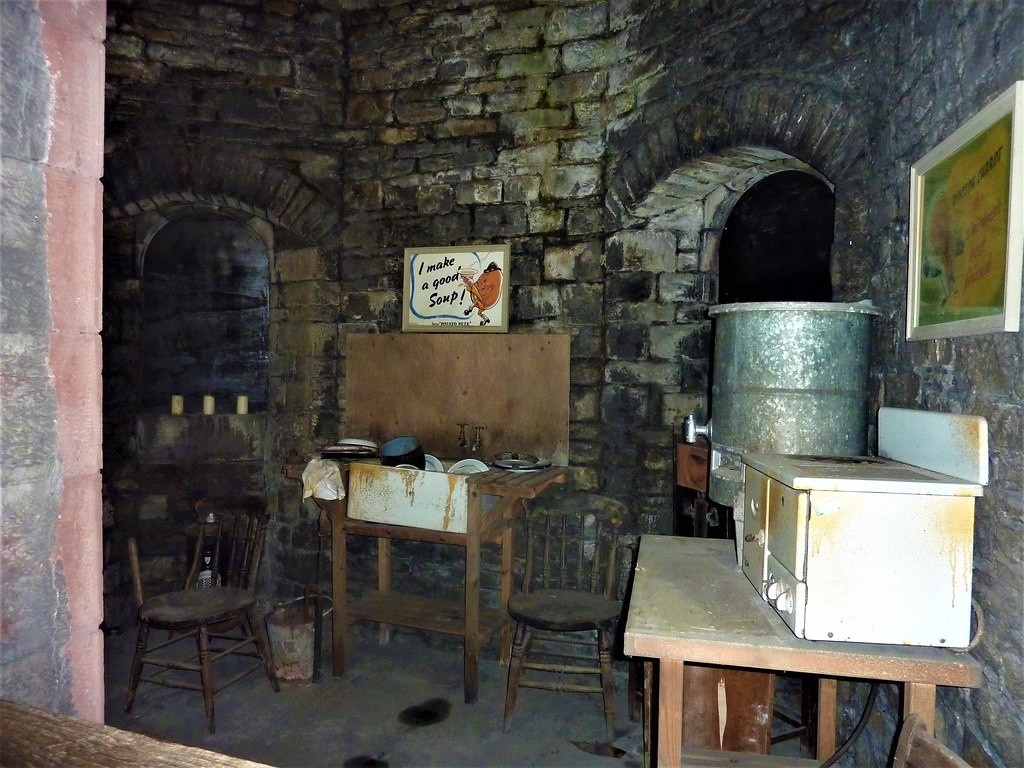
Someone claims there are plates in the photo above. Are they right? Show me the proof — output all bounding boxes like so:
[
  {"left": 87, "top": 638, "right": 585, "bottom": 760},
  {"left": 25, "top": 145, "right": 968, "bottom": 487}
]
[
  {"left": 447, "top": 459, "right": 489, "bottom": 474},
  {"left": 425, "top": 454, "right": 444, "bottom": 472},
  {"left": 338, "top": 439, "right": 378, "bottom": 448}
]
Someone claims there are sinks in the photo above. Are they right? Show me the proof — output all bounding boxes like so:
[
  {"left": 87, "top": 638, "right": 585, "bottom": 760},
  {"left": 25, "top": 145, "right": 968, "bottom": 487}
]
[{"left": 346, "top": 453, "right": 508, "bottom": 533}]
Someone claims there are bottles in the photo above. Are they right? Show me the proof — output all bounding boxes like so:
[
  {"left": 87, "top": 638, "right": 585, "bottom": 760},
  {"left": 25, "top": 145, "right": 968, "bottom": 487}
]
[{"left": 195, "top": 552, "right": 221, "bottom": 587}]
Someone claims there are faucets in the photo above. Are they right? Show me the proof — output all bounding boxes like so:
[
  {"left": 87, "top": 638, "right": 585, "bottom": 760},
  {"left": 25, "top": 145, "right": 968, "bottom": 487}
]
[
  {"left": 472, "top": 424, "right": 484, "bottom": 451},
  {"left": 452, "top": 422, "right": 468, "bottom": 453}
]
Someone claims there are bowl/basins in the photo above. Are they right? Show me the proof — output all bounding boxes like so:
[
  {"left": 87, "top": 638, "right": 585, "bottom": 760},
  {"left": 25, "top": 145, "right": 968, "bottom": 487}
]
[{"left": 378, "top": 436, "right": 425, "bottom": 469}]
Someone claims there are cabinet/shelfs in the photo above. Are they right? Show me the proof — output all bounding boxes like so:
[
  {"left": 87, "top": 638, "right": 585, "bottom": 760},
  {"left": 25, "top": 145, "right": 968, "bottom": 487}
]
[
  {"left": 741, "top": 456, "right": 973, "bottom": 649},
  {"left": 283, "top": 464, "right": 568, "bottom": 703}
]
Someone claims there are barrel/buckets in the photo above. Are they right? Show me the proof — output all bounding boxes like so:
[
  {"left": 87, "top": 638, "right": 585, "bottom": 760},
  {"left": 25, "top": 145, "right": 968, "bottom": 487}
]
[
  {"left": 709, "top": 302, "right": 883, "bottom": 506},
  {"left": 265, "top": 594, "right": 333, "bottom": 679}
]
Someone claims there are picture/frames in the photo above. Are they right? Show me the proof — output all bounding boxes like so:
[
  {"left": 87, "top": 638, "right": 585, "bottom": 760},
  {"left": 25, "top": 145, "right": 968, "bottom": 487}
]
[
  {"left": 402, "top": 245, "right": 511, "bottom": 333},
  {"left": 906, "top": 79, "right": 1024, "bottom": 342}
]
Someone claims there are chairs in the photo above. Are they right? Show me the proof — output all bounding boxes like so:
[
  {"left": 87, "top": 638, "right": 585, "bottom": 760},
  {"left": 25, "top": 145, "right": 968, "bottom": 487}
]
[
  {"left": 502, "top": 491, "right": 631, "bottom": 741},
  {"left": 124, "top": 496, "right": 281, "bottom": 735}
]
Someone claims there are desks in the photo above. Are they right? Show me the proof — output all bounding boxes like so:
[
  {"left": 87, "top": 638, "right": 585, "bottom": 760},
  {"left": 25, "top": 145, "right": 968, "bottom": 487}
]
[{"left": 624, "top": 534, "right": 983, "bottom": 768}]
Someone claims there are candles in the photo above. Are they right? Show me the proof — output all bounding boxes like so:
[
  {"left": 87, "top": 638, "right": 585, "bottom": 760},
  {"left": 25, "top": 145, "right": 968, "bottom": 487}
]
[
  {"left": 171, "top": 395, "right": 184, "bottom": 415},
  {"left": 237, "top": 396, "right": 249, "bottom": 415},
  {"left": 204, "top": 393, "right": 215, "bottom": 415}
]
